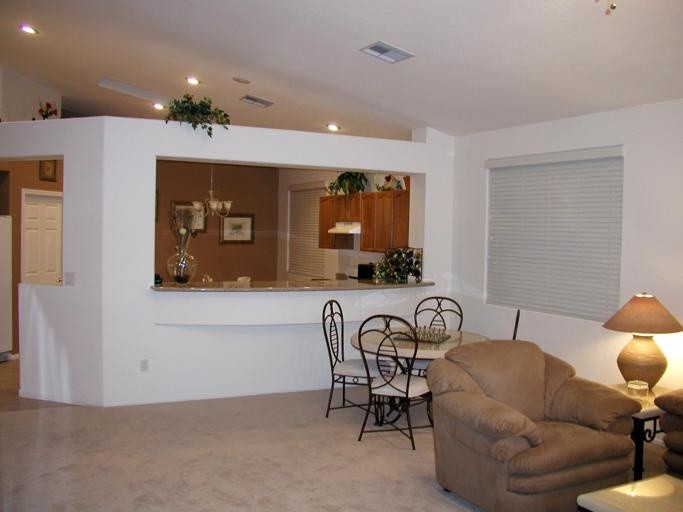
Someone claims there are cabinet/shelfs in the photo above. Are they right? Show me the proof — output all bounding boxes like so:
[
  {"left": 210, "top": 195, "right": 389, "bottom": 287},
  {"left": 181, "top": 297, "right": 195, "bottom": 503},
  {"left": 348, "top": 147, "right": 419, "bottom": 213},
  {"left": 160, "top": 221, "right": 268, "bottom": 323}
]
[{"left": 318, "top": 190, "right": 409, "bottom": 255}]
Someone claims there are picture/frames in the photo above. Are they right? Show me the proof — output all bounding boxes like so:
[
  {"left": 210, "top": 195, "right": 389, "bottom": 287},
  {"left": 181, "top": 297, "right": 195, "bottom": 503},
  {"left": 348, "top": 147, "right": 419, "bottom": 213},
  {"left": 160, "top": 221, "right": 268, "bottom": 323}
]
[
  {"left": 217, "top": 212, "right": 255, "bottom": 245},
  {"left": 168, "top": 198, "right": 208, "bottom": 234},
  {"left": 36, "top": 159, "right": 57, "bottom": 183}
]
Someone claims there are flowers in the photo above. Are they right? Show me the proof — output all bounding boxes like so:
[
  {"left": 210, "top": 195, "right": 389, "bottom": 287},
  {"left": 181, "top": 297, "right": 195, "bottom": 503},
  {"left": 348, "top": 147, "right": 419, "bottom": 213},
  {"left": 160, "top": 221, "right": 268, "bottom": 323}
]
[{"left": 33, "top": 100, "right": 59, "bottom": 120}]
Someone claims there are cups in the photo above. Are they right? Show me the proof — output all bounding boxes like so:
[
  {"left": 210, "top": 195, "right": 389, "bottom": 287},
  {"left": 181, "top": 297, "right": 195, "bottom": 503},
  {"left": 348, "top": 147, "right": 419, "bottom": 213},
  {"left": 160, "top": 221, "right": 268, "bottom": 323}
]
[{"left": 626, "top": 380, "right": 648, "bottom": 413}]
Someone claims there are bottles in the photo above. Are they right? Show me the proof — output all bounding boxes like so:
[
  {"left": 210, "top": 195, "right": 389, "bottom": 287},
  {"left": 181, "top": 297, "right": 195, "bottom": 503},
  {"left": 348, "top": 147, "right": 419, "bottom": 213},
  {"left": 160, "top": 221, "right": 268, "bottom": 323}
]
[{"left": 398, "top": 325, "right": 448, "bottom": 341}]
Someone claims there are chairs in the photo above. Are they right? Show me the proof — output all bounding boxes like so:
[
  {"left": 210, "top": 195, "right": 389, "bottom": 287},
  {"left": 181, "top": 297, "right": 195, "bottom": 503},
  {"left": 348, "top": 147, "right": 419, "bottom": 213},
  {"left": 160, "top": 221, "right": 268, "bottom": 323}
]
[
  {"left": 355, "top": 314, "right": 434, "bottom": 453},
  {"left": 651, "top": 385, "right": 682, "bottom": 479},
  {"left": 402, "top": 295, "right": 464, "bottom": 379},
  {"left": 318, "top": 298, "right": 388, "bottom": 428},
  {"left": 511, "top": 306, "right": 520, "bottom": 339},
  {"left": 423, "top": 335, "right": 641, "bottom": 512}
]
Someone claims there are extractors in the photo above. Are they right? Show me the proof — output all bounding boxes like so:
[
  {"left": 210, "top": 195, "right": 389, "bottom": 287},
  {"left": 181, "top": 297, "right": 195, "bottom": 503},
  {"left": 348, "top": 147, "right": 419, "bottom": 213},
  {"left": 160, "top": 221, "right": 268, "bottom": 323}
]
[{"left": 327, "top": 220, "right": 362, "bottom": 234}]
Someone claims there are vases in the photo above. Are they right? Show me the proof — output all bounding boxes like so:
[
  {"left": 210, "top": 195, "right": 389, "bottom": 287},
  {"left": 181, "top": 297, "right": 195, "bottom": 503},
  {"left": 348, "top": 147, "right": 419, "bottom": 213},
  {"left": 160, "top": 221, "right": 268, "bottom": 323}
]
[{"left": 164, "top": 230, "right": 199, "bottom": 286}]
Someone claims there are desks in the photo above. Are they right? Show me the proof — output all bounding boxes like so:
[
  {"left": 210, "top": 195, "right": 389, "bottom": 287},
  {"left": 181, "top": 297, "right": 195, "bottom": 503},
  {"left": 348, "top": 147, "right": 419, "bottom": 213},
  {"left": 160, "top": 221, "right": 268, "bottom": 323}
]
[
  {"left": 346, "top": 325, "right": 489, "bottom": 427},
  {"left": 603, "top": 379, "right": 676, "bottom": 483},
  {"left": 573, "top": 470, "right": 682, "bottom": 512}
]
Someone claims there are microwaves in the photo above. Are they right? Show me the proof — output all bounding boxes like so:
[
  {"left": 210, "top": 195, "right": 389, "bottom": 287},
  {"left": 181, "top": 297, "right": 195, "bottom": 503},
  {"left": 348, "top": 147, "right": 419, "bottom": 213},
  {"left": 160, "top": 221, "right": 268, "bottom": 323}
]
[{"left": 358, "top": 262, "right": 378, "bottom": 280}]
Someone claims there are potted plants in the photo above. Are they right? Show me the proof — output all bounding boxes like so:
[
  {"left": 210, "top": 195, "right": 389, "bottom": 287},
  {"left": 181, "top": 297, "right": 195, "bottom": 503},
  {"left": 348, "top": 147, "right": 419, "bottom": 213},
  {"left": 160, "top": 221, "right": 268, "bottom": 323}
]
[
  {"left": 161, "top": 91, "right": 232, "bottom": 139},
  {"left": 329, "top": 169, "right": 370, "bottom": 194}
]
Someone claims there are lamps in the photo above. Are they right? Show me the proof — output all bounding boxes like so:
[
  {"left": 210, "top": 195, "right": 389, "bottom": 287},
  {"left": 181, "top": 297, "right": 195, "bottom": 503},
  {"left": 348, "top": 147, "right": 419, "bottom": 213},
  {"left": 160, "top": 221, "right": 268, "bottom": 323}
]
[
  {"left": 600, "top": 291, "right": 682, "bottom": 397},
  {"left": 191, "top": 164, "right": 233, "bottom": 219}
]
